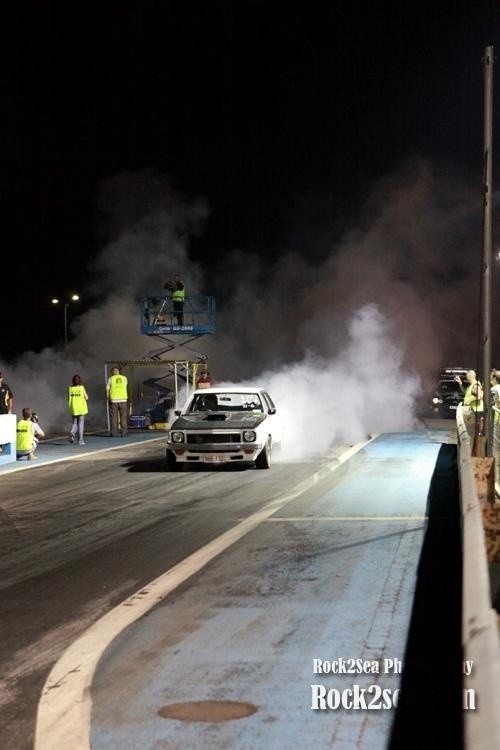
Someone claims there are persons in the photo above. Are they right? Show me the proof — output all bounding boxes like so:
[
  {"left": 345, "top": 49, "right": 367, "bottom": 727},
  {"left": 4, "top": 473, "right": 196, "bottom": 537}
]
[
  {"left": 0, "top": 371, "right": 14, "bottom": 453},
  {"left": 67, "top": 375, "right": 89, "bottom": 445},
  {"left": 170, "top": 274, "right": 186, "bottom": 326},
  {"left": 489, "top": 368, "right": 500, "bottom": 411},
  {"left": 106, "top": 367, "right": 129, "bottom": 437},
  {"left": 454, "top": 370, "right": 485, "bottom": 421},
  {"left": 16, "top": 407, "right": 45, "bottom": 461},
  {"left": 196, "top": 368, "right": 214, "bottom": 389}
]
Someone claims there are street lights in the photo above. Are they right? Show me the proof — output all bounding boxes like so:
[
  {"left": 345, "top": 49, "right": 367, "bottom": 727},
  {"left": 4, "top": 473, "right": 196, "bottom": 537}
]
[{"left": 50, "top": 292, "right": 81, "bottom": 346}]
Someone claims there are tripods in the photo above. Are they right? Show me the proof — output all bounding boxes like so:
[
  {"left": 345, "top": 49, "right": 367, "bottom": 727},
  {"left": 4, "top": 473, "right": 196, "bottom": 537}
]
[{"left": 154, "top": 291, "right": 176, "bottom": 326}]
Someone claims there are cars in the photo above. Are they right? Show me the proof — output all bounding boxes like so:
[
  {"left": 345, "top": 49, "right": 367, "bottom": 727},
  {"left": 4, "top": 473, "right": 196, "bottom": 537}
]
[{"left": 162, "top": 385, "right": 283, "bottom": 472}]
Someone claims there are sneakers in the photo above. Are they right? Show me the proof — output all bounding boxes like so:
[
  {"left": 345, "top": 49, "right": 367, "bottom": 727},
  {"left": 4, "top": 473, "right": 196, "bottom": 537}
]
[
  {"left": 28, "top": 454, "right": 37, "bottom": 460},
  {"left": 69, "top": 432, "right": 86, "bottom": 445}
]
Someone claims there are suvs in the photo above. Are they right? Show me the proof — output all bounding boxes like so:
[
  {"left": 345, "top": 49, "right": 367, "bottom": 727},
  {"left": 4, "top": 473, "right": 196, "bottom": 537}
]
[{"left": 428, "top": 365, "right": 474, "bottom": 418}]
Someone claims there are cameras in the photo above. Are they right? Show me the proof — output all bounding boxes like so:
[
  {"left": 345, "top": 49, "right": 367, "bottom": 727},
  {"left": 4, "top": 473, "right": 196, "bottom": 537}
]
[{"left": 32, "top": 413, "right": 38, "bottom": 421}]
